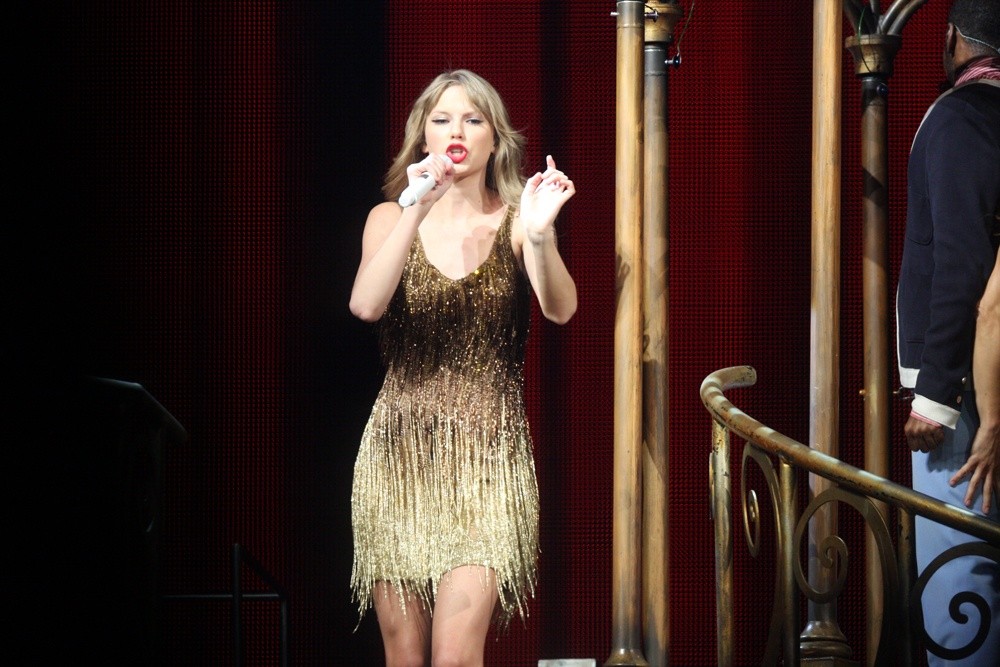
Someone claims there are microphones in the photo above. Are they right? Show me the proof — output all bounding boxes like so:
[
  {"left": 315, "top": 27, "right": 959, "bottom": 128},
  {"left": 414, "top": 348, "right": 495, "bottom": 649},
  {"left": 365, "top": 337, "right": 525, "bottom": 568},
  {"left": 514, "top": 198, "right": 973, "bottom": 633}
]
[{"left": 398, "top": 154, "right": 454, "bottom": 208}]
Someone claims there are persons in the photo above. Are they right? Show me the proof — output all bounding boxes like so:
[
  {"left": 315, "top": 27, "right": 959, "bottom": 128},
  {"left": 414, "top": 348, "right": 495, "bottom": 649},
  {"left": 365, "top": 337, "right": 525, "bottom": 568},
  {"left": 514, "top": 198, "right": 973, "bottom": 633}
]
[
  {"left": 948, "top": 246, "right": 1000, "bottom": 514},
  {"left": 348, "top": 69, "right": 579, "bottom": 667},
  {"left": 896, "top": 0, "right": 1000, "bottom": 667}
]
